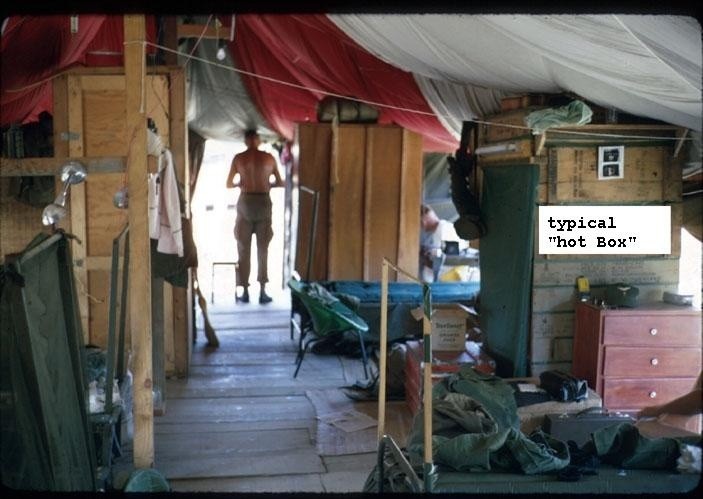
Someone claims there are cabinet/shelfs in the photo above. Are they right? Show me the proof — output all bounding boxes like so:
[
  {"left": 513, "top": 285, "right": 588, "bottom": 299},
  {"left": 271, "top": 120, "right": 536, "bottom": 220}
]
[
  {"left": 401, "top": 337, "right": 496, "bottom": 420},
  {"left": 571, "top": 301, "right": 703, "bottom": 418}
]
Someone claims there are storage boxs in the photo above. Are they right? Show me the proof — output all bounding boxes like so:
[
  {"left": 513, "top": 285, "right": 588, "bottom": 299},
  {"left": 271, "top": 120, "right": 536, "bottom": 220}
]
[{"left": 409, "top": 299, "right": 481, "bottom": 353}]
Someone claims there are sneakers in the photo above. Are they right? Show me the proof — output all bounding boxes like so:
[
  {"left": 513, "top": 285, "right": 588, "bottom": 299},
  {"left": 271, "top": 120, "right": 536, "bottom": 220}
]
[
  {"left": 259, "top": 292, "right": 273, "bottom": 303},
  {"left": 236, "top": 293, "right": 250, "bottom": 302}
]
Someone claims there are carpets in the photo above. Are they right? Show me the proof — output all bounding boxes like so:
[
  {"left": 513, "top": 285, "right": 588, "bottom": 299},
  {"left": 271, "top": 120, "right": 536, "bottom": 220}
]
[{"left": 305, "top": 384, "right": 411, "bottom": 455}]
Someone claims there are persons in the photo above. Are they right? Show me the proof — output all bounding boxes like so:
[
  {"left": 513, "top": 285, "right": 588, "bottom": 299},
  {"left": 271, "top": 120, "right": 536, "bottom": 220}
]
[
  {"left": 226, "top": 130, "right": 288, "bottom": 303},
  {"left": 421, "top": 203, "right": 468, "bottom": 247},
  {"left": 633, "top": 369, "right": 703, "bottom": 441}
]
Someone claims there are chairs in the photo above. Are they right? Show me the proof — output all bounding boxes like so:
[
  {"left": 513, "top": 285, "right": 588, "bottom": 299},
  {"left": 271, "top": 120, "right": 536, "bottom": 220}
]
[{"left": 288, "top": 280, "right": 372, "bottom": 381}]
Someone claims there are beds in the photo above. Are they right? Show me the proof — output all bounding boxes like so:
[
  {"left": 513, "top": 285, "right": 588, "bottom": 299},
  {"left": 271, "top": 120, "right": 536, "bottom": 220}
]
[
  {"left": 377, "top": 433, "right": 703, "bottom": 499},
  {"left": 311, "top": 277, "right": 483, "bottom": 353}
]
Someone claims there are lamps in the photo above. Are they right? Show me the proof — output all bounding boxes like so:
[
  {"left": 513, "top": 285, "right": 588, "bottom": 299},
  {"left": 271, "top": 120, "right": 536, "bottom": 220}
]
[{"left": 36, "top": 159, "right": 91, "bottom": 228}]
